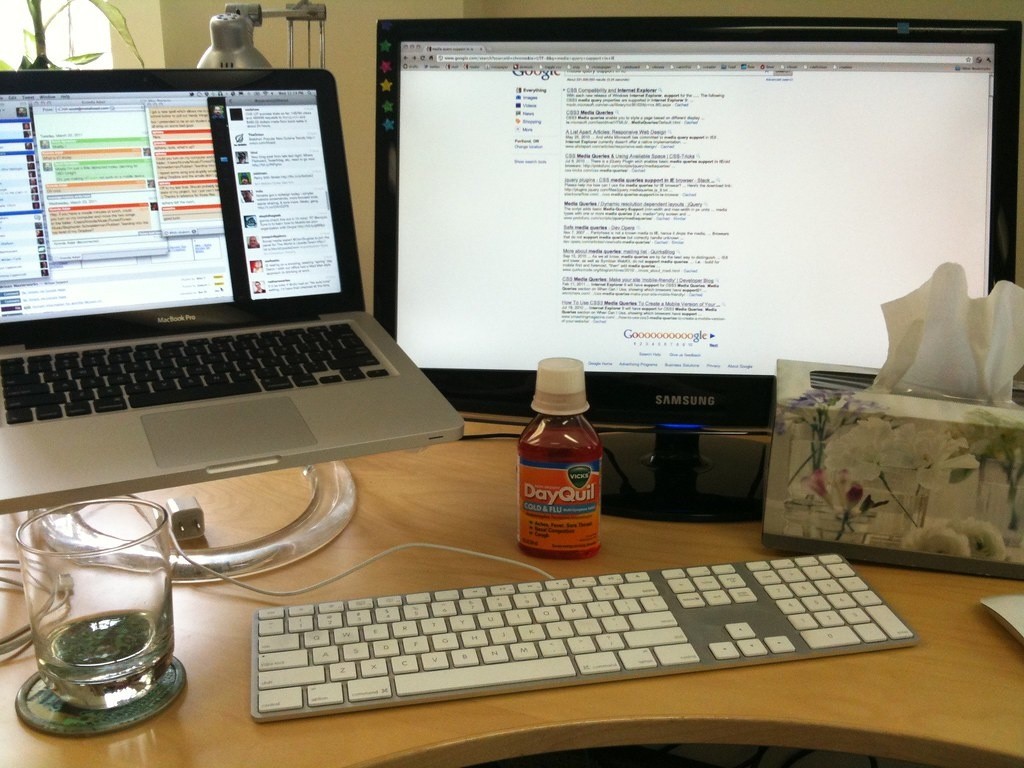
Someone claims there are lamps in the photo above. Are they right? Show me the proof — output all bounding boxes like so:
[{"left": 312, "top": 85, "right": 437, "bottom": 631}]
[{"left": 197, "top": 0, "right": 327, "bottom": 68}]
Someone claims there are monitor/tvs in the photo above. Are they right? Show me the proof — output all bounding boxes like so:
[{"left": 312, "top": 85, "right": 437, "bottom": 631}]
[{"left": 377, "top": 12, "right": 1024, "bottom": 522}]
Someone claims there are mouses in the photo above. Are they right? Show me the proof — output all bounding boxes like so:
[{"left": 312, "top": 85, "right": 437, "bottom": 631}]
[{"left": 981, "top": 594, "right": 1024, "bottom": 646}]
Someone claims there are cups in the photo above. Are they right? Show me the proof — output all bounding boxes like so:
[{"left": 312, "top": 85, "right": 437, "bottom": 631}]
[{"left": 16, "top": 498, "right": 175, "bottom": 710}]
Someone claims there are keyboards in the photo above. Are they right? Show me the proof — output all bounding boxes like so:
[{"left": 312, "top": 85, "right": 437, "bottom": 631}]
[{"left": 252, "top": 552, "right": 920, "bottom": 727}]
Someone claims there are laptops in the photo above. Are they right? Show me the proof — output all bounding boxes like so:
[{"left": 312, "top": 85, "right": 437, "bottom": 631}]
[{"left": 0, "top": 68, "right": 463, "bottom": 513}]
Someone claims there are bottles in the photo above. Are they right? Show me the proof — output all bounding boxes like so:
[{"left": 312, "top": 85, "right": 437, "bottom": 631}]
[{"left": 518, "top": 357, "right": 602, "bottom": 558}]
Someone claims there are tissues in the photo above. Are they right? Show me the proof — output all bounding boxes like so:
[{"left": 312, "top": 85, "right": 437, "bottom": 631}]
[{"left": 760, "top": 261, "right": 1024, "bottom": 579}]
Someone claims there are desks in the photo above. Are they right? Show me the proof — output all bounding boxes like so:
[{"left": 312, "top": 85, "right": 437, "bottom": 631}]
[{"left": 0, "top": 411, "right": 1024, "bottom": 768}]
